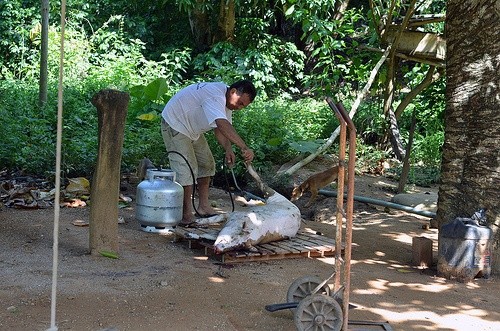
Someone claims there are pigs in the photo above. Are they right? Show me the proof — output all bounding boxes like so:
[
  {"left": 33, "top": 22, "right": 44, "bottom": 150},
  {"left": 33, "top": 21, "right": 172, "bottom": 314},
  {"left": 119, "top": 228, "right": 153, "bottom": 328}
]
[{"left": 183, "top": 161, "right": 301, "bottom": 256}]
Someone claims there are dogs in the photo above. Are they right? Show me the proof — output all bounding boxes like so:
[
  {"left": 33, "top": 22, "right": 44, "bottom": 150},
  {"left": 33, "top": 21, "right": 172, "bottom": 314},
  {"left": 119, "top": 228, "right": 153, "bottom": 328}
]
[{"left": 289, "top": 162, "right": 347, "bottom": 208}]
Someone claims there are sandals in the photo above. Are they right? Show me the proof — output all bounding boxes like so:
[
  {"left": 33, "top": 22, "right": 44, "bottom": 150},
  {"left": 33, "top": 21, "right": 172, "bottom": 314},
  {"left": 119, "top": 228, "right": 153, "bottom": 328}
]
[
  {"left": 182, "top": 219, "right": 197, "bottom": 224},
  {"left": 195, "top": 207, "right": 223, "bottom": 218}
]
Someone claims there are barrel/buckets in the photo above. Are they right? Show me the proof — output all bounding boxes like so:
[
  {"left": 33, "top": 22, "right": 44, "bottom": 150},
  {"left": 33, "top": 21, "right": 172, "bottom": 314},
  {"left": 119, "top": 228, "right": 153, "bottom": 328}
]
[{"left": 435, "top": 212, "right": 492, "bottom": 283}]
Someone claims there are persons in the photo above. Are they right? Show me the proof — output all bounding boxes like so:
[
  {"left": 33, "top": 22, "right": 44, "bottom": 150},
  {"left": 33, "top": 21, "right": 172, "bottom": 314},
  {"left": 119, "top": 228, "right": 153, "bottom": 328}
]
[{"left": 159, "top": 79, "right": 259, "bottom": 230}]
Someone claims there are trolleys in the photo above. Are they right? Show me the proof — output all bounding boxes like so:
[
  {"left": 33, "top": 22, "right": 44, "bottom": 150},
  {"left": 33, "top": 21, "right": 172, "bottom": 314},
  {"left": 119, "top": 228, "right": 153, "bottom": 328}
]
[{"left": 264, "top": 96, "right": 393, "bottom": 331}]
